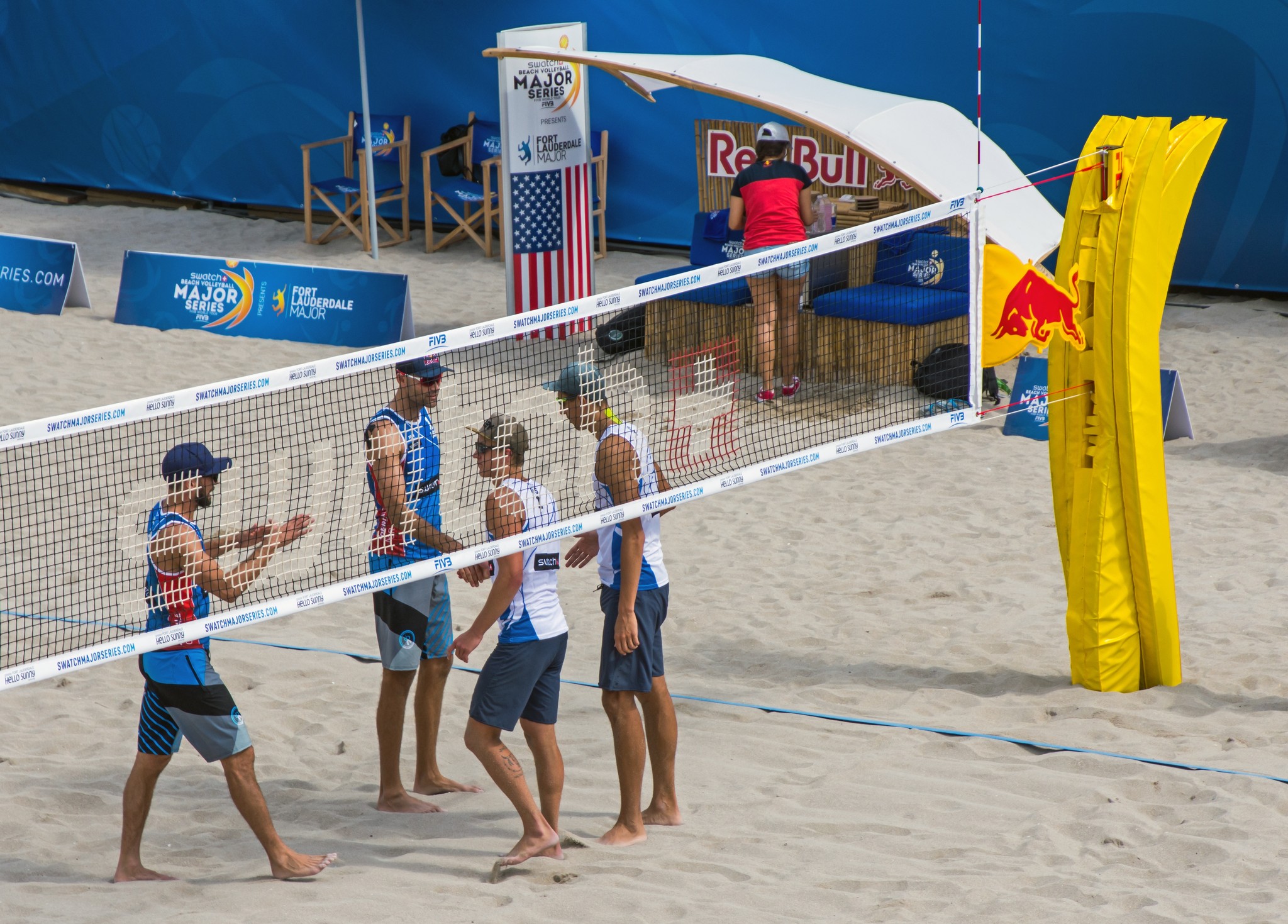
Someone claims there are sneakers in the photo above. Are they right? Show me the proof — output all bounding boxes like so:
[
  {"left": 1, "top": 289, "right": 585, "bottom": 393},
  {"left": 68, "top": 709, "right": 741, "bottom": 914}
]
[
  {"left": 782, "top": 375, "right": 800, "bottom": 398},
  {"left": 755, "top": 386, "right": 774, "bottom": 402}
]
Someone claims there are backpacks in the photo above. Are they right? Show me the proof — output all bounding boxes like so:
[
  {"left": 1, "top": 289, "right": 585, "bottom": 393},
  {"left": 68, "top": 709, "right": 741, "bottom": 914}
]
[{"left": 436, "top": 116, "right": 477, "bottom": 175}]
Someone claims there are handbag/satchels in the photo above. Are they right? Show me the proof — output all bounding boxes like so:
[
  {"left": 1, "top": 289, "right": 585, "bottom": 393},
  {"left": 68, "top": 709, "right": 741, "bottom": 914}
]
[{"left": 910, "top": 343, "right": 1000, "bottom": 406}]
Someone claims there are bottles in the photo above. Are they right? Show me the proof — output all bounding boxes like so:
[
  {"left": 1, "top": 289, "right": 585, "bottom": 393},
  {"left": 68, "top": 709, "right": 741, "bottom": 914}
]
[
  {"left": 814, "top": 195, "right": 826, "bottom": 232},
  {"left": 804, "top": 201, "right": 814, "bottom": 233},
  {"left": 822, "top": 194, "right": 832, "bottom": 230}
]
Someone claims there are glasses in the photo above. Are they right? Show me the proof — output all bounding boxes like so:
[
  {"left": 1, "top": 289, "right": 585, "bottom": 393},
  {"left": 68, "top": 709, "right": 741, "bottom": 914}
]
[
  {"left": 475, "top": 442, "right": 503, "bottom": 453},
  {"left": 397, "top": 369, "right": 443, "bottom": 387},
  {"left": 201, "top": 474, "right": 218, "bottom": 483},
  {"left": 555, "top": 395, "right": 576, "bottom": 406}
]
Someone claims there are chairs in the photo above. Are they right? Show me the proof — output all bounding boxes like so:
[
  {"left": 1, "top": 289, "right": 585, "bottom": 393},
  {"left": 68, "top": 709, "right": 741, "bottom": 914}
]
[
  {"left": 420, "top": 111, "right": 503, "bottom": 258},
  {"left": 494, "top": 129, "right": 609, "bottom": 261},
  {"left": 300, "top": 111, "right": 411, "bottom": 252},
  {"left": 634, "top": 206, "right": 969, "bottom": 391}
]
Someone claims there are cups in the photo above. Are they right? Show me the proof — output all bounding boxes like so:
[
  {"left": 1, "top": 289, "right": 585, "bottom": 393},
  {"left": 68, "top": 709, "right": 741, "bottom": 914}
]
[{"left": 832, "top": 204, "right": 837, "bottom": 228}]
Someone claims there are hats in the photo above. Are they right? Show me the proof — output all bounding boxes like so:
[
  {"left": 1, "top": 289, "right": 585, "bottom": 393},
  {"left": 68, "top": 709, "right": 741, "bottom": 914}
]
[
  {"left": 463, "top": 412, "right": 529, "bottom": 455},
  {"left": 543, "top": 361, "right": 605, "bottom": 401},
  {"left": 395, "top": 354, "right": 455, "bottom": 378},
  {"left": 162, "top": 442, "right": 232, "bottom": 483},
  {"left": 757, "top": 122, "right": 793, "bottom": 150}
]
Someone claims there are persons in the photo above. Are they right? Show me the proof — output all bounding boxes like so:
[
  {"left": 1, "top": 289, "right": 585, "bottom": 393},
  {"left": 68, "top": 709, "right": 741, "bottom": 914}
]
[
  {"left": 364, "top": 352, "right": 490, "bottom": 815},
  {"left": 729, "top": 121, "right": 815, "bottom": 403},
  {"left": 112, "top": 441, "right": 338, "bottom": 883},
  {"left": 542, "top": 362, "right": 682, "bottom": 845},
  {"left": 445, "top": 412, "right": 569, "bottom": 865}
]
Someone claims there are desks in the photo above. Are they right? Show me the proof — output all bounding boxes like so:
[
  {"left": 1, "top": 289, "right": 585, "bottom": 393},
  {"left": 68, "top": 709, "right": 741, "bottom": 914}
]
[{"left": 809, "top": 195, "right": 909, "bottom": 306}]
